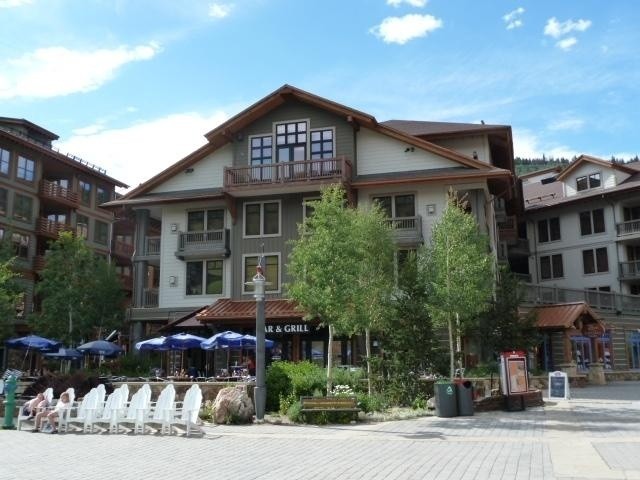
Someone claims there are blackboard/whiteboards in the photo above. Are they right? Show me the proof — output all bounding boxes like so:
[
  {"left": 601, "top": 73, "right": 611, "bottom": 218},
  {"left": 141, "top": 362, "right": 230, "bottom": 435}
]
[{"left": 548, "top": 375, "right": 567, "bottom": 400}]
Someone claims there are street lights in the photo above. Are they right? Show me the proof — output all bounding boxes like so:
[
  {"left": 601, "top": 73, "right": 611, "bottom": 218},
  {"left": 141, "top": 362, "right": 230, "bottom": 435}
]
[{"left": 251, "top": 253, "right": 268, "bottom": 423}]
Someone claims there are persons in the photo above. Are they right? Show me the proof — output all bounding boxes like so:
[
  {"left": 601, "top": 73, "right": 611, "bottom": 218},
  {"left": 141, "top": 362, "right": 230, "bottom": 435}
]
[
  {"left": 247, "top": 357, "right": 256, "bottom": 376},
  {"left": 31, "top": 391, "right": 69, "bottom": 434},
  {"left": 1, "top": 362, "right": 22, "bottom": 404},
  {"left": 186, "top": 364, "right": 199, "bottom": 378},
  {"left": 25, "top": 393, "right": 47, "bottom": 429},
  {"left": 37, "top": 363, "right": 62, "bottom": 379}
]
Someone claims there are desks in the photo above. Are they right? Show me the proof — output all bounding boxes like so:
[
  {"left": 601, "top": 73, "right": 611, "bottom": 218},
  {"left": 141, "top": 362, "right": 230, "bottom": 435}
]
[{"left": 108, "top": 375, "right": 256, "bottom": 382}]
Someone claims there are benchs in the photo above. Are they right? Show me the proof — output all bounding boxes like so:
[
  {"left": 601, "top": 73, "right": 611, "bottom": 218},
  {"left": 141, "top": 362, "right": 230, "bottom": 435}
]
[{"left": 299, "top": 396, "right": 362, "bottom": 424}]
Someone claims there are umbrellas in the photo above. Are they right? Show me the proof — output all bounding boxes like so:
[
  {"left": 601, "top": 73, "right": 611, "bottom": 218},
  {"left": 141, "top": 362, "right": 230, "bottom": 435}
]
[
  {"left": 227, "top": 334, "right": 274, "bottom": 368},
  {"left": 42, "top": 347, "right": 84, "bottom": 375},
  {"left": 200, "top": 331, "right": 243, "bottom": 375},
  {"left": 161, "top": 332, "right": 209, "bottom": 371},
  {"left": 4, "top": 333, "right": 61, "bottom": 372},
  {"left": 76, "top": 339, "right": 125, "bottom": 374},
  {"left": 134, "top": 336, "right": 171, "bottom": 375}
]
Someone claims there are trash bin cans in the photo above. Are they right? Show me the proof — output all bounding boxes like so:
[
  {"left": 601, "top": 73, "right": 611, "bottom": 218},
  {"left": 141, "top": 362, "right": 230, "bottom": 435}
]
[
  {"left": 454, "top": 380, "right": 474, "bottom": 416},
  {"left": 434, "top": 383, "right": 456, "bottom": 418}
]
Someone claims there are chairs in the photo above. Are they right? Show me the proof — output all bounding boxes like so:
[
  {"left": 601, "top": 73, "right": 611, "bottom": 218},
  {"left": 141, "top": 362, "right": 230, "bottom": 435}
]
[{"left": 16, "top": 384, "right": 204, "bottom": 437}]
